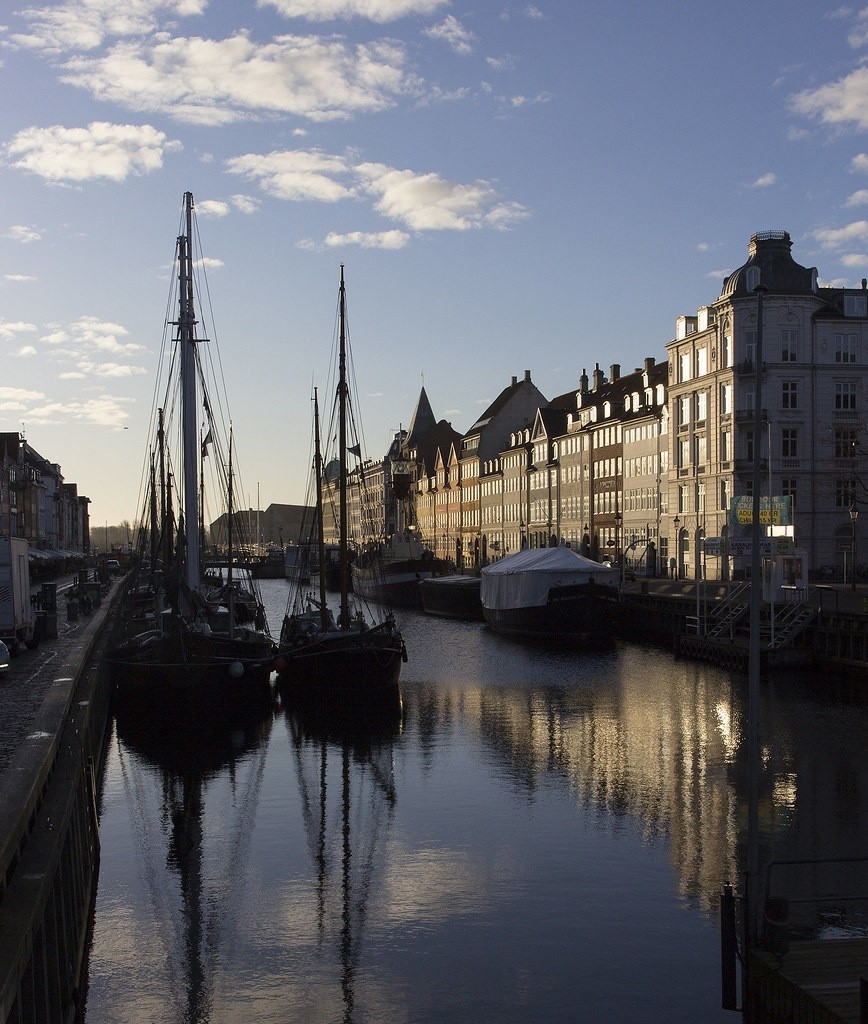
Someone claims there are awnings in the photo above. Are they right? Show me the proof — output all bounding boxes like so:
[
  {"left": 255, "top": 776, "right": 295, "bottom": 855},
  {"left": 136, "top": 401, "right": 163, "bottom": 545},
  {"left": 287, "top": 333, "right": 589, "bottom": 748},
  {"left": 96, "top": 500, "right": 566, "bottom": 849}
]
[{"left": 28, "top": 547, "right": 88, "bottom": 561}]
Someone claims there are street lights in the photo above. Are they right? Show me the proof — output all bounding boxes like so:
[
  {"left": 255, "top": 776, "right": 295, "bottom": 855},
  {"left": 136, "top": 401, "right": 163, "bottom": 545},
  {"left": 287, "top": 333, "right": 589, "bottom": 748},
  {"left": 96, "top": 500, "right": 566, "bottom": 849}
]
[
  {"left": 613, "top": 512, "right": 623, "bottom": 566},
  {"left": 672, "top": 515, "right": 680, "bottom": 583},
  {"left": 475, "top": 531, "right": 481, "bottom": 572},
  {"left": 583, "top": 523, "right": 589, "bottom": 556},
  {"left": 848, "top": 500, "right": 861, "bottom": 593},
  {"left": 442, "top": 533, "right": 447, "bottom": 560},
  {"left": 519, "top": 521, "right": 526, "bottom": 551}
]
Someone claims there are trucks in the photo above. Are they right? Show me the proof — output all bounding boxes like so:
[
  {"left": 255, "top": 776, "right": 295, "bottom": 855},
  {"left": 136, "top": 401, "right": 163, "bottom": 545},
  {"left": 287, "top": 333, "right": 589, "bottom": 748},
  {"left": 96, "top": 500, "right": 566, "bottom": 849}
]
[{"left": 0, "top": 537, "right": 33, "bottom": 655}]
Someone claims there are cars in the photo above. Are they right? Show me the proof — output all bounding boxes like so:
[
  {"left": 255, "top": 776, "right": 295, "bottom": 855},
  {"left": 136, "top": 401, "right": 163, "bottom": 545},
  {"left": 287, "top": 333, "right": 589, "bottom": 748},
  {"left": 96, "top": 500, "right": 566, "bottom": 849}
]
[{"left": 107, "top": 559, "right": 119, "bottom": 571}]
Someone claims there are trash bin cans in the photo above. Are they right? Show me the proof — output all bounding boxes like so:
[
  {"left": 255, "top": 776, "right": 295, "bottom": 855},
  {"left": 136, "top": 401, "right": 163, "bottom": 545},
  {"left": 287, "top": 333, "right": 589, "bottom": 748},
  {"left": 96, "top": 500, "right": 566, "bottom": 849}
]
[
  {"left": 78, "top": 582, "right": 102, "bottom": 608},
  {"left": 42, "top": 583, "right": 57, "bottom": 610},
  {"left": 79, "top": 569, "right": 87, "bottom": 582},
  {"left": 642, "top": 582, "right": 648, "bottom": 593}
]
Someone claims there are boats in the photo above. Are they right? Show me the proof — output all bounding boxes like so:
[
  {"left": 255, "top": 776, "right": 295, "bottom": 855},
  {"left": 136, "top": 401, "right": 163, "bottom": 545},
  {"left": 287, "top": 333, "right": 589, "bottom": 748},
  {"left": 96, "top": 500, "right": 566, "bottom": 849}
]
[
  {"left": 480, "top": 543, "right": 624, "bottom": 643},
  {"left": 283, "top": 544, "right": 316, "bottom": 584},
  {"left": 310, "top": 542, "right": 356, "bottom": 593},
  {"left": 250, "top": 547, "right": 285, "bottom": 580},
  {"left": 417, "top": 574, "right": 486, "bottom": 620}
]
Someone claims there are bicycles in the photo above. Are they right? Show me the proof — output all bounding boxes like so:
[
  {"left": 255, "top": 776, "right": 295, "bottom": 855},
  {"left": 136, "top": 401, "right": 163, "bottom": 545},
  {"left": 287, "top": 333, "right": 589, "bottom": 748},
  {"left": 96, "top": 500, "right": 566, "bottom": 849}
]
[{"left": 77, "top": 585, "right": 94, "bottom": 617}]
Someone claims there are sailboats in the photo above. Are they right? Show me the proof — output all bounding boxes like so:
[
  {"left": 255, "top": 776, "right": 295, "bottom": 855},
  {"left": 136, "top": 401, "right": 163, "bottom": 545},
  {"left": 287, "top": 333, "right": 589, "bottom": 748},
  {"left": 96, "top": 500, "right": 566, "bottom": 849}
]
[
  {"left": 102, "top": 184, "right": 280, "bottom": 713},
  {"left": 200, "top": 424, "right": 258, "bottom": 623},
  {"left": 265, "top": 257, "right": 410, "bottom": 703},
  {"left": 350, "top": 422, "right": 439, "bottom": 608}
]
